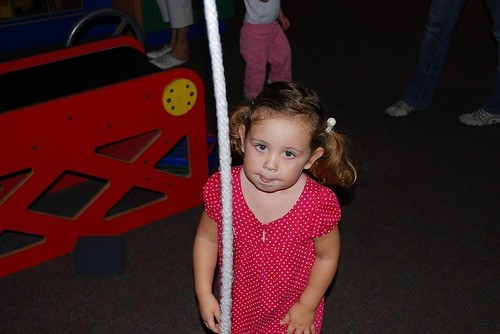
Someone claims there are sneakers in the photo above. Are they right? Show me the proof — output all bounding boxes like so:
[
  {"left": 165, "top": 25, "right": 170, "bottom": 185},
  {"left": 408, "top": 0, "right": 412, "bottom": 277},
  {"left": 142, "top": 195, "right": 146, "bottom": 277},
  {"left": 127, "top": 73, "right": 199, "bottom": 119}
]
[
  {"left": 149, "top": 53, "right": 190, "bottom": 69},
  {"left": 459, "top": 108, "right": 500, "bottom": 126},
  {"left": 147, "top": 45, "right": 172, "bottom": 59},
  {"left": 385, "top": 100, "right": 414, "bottom": 116}
]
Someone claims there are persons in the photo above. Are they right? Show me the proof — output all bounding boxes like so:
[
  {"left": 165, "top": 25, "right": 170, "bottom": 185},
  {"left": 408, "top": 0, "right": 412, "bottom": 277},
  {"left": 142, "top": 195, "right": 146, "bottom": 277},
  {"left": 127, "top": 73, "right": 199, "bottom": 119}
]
[
  {"left": 239, "top": 0, "right": 292, "bottom": 102},
  {"left": 145, "top": 0, "right": 194, "bottom": 70},
  {"left": 193, "top": 83, "right": 357, "bottom": 334},
  {"left": 388, "top": 0, "right": 500, "bottom": 126}
]
